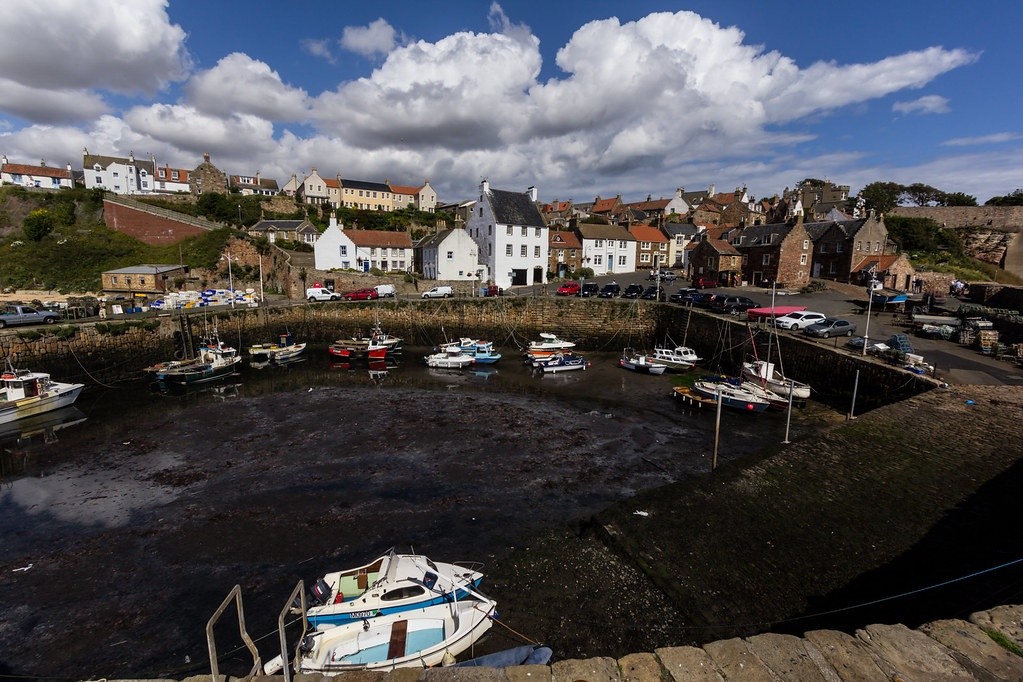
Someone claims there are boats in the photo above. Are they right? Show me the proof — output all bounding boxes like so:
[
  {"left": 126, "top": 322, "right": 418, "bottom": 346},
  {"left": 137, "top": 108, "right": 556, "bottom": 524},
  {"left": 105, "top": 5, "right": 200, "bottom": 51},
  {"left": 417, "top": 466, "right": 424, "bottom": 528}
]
[
  {"left": 293, "top": 547, "right": 484, "bottom": 632},
  {"left": 424, "top": 327, "right": 504, "bottom": 369},
  {"left": 3, "top": 405, "right": 90, "bottom": 446},
  {"left": 124, "top": 312, "right": 307, "bottom": 387},
  {"left": 439, "top": 641, "right": 551, "bottom": 666},
  {"left": 614, "top": 293, "right": 813, "bottom": 415},
  {"left": 324, "top": 299, "right": 405, "bottom": 368},
  {"left": 867, "top": 290, "right": 908, "bottom": 303},
  {"left": 293, "top": 561, "right": 497, "bottom": 676},
  {"left": 0, "top": 356, "right": 85, "bottom": 422},
  {"left": 524, "top": 331, "right": 596, "bottom": 375}
]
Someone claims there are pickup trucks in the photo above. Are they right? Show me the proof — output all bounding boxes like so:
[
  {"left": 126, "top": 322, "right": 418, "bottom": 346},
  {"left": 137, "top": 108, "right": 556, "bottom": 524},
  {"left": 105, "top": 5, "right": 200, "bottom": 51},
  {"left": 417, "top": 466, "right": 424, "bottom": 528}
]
[{"left": 0, "top": 304, "right": 61, "bottom": 329}]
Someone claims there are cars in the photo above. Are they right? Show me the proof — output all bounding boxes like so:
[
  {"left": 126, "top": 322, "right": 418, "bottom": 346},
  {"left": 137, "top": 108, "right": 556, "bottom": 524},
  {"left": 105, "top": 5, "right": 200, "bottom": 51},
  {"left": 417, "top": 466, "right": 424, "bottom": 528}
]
[
  {"left": 623, "top": 284, "right": 645, "bottom": 299},
  {"left": 683, "top": 293, "right": 704, "bottom": 306},
  {"left": 660, "top": 270, "right": 679, "bottom": 282},
  {"left": 670, "top": 288, "right": 700, "bottom": 303},
  {"left": 599, "top": 283, "right": 622, "bottom": 298},
  {"left": 345, "top": 287, "right": 379, "bottom": 299},
  {"left": 556, "top": 282, "right": 581, "bottom": 296},
  {"left": 698, "top": 294, "right": 719, "bottom": 309},
  {"left": 576, "top": 282, "right": 600, "bottom": 298},
  {"left": 640, "top": 286, "right": 666, "bottom": 301},
  {"left": 692, "top": 273, "right": 721, "bottom": 289},
  {"left": 773, "top": 309, "right": 828, "bottom": 331},
  {"left": 803, "top": 318, "right": 857, "bottom": 339}
]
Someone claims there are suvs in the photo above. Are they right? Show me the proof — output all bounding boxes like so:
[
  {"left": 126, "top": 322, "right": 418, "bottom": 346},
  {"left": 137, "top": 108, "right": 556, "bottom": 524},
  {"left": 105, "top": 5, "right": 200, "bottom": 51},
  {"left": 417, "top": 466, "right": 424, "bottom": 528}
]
[
  {"left": 421, "top": 285, "right": 455, "bottom": 299},
  {"left": 713, "top": 294, "right": 762, "bottom": 314}
]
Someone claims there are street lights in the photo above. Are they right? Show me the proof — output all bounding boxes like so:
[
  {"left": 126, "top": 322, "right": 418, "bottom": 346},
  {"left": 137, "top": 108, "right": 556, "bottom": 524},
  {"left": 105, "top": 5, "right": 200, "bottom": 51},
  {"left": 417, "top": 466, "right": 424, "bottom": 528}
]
[{"left": 220, "top": 251, "right": 239, "bottom": 309}]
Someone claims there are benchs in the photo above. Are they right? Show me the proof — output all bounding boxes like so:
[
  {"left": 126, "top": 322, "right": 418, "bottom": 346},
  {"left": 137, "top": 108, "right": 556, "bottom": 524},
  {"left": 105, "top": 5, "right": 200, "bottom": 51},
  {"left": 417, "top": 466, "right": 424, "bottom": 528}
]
[{"left": 387, "top": 620, "right": 408, "bottom": 660}]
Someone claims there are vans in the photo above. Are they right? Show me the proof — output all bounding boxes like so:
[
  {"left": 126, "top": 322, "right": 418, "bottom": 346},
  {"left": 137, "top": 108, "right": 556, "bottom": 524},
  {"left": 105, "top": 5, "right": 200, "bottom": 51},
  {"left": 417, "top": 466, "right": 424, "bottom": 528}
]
[
  {"left": 305, "top": 287, "right": 342, "bottom": 303},
  {"left": 375, "top": 284, "right": 396, "bottom": 298}
]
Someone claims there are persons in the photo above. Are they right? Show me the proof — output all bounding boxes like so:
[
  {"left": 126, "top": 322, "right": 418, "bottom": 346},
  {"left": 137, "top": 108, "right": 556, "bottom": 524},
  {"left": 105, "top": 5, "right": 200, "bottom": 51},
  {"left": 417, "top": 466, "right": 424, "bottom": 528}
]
[
  {"left": 98, "top": 300, "right": 108, "bottom": 320},
  {"left": 649, "top": 267, "right": 654, "bottom": 281},
  {"left": 949, "top": 279, "right": 969, "bottom": 298},
  {"left": 612, "top": 278, "right": 616, "bottom": 283},
  {"left": 670, "top": 277, "right": 673, "bottom": 282},
  {"left": 660, "top": 276, "right": 667, "bottom": 284},
  {"left": 913, "top": 278, "right": 923, "bottom": 293},
  {"left": 654, "top": 268, "right": 659, "bottom": 278}
]
